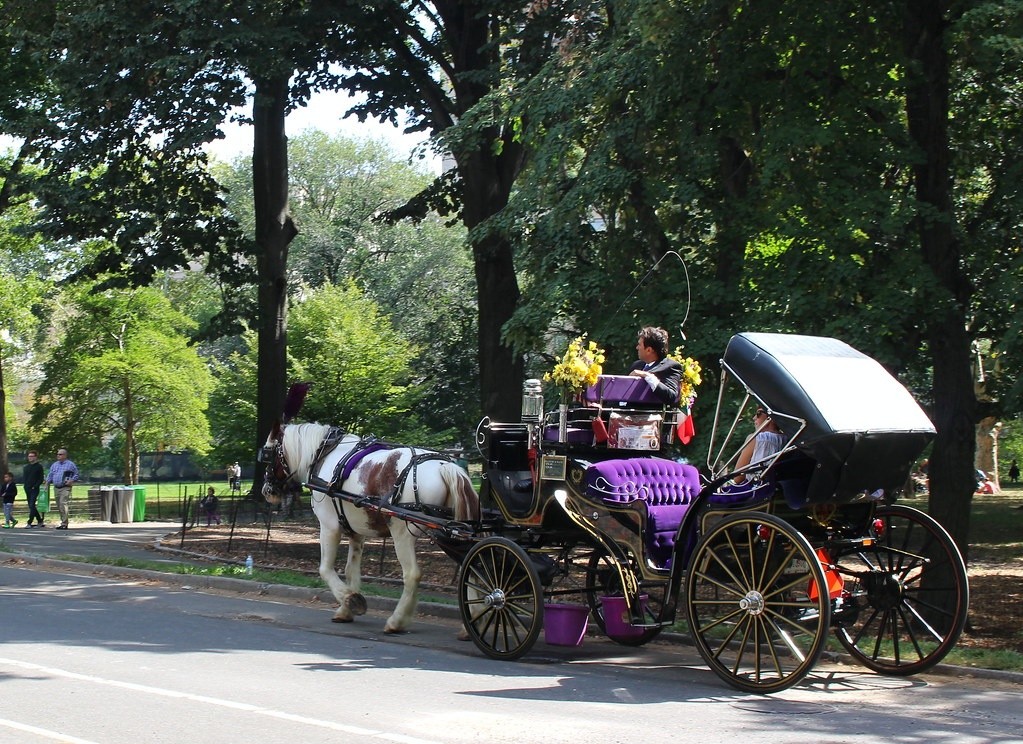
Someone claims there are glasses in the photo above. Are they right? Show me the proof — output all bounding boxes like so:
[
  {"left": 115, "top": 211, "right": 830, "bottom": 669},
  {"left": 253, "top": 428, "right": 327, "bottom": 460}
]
[
  {"left": 57, "top": 454, "right": 64, "bottom": 455},
  {"left": 756, "top": 410, "right": 769, "bottom": 417}
]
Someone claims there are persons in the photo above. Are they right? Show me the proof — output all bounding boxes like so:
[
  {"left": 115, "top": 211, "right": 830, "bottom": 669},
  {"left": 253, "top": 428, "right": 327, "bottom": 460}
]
[
  {"left": 201, "top": 488, "right": 222, "bottom": 526},
  {"left": 290, "top": 481, "right": 304, "bottom": 516},
  {"left": 228, "top": 462, "right": 241, "bottom": 492},
  {"left": 978, "top": 478, "right": 996, "bottom": 494},
  {"left": 733, "top": 404, "right": 788, "bottom": 485},
  {"left": 921, "top": 459, "right": 928, "bottom": 473},
  {"left": 0, "top": 473, "right": 18, "bottom": 528},
  {"left": 451, "top": 453, "right": 468, "bottom": 473},
  {"left": 44, "top": 449, "right": 78, "bottom": 530},
  {"left": 1008, "top": 460, "right": 1020, "bottom": 482},
  {"left": 626, "top": 327, "right": 683, "bottom": 405},
  {"left": 23, "top": 450, "right": 44, "bottom": 529}
]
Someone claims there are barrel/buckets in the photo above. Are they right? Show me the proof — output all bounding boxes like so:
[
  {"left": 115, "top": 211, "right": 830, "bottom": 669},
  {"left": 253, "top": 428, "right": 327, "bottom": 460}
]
[
  {"left": 599, "top": 594, "right": 648, "bottom": 637},
  {"left": 544, "top": 604, "right": 592, "bottom": 647}
]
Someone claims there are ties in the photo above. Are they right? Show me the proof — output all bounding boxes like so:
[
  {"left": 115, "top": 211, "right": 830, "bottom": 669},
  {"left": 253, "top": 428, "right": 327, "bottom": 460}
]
[{"left": 643, "top": 365, "right": 649, "bottom": 371}]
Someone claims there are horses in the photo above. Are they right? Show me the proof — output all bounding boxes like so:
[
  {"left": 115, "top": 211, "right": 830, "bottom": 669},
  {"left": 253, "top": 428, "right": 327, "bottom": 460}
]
[{"left": 261, "top": 420, "right": 485, "bottom": 640}]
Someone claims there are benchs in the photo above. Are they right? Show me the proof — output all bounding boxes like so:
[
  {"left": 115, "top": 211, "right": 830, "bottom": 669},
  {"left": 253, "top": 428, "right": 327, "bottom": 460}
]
[
  {"left": 544, "top": 373, "right": 681, "bottom": 444},
  {"left": 589, "top": 458, "right": 699, "bottom": 541},
  {"left": 711, "top": 434, "right": 792, "bottom": 507}
]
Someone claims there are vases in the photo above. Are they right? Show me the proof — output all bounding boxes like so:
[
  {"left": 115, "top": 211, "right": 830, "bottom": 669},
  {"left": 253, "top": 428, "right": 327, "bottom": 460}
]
[
  {"left": 559, "top": 403, "right": 568, "bottom": 442},
  {"left": 667, "top": 408, "right": 677, "bottom": 444}
]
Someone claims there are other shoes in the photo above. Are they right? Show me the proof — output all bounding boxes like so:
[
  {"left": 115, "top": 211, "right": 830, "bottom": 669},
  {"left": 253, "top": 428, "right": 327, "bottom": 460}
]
[
  {"left": 33, "top": 522, "right": 43, "bottom": 529},
  {"left": 25, "top": 524, "right": 31, "bottom": 528},
  {"left": 4, "top": 524, "right": 10, "bottom": 529},
  {"left": 11, "top": 519, "right": 19, "bottom": 528},
  {"left": 56, "top": 524, "right": 68, "bottom": 529}
]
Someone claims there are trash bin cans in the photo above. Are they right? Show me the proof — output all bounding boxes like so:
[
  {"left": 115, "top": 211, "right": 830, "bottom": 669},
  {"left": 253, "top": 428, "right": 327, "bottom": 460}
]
[
  {"left": 88, "top": 486, "right": 112, "bottom": 522},
  {"left": 119, "top": 485, "right": 134, "bottom": 523},
  {"left": 108, "top": 485, "right": 123, "bottom": 523},
  {"left": 128, "top": 485, "right": 146, "bottom": 521}
]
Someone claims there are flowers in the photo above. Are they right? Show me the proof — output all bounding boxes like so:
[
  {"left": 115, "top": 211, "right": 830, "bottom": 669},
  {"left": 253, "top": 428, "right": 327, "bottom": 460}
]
[
  {"left": 543, "top": 332, "right": 604, "bottom": 402},
  {"left": 668, "top": 344, "right": 702, "bottom": 410}
]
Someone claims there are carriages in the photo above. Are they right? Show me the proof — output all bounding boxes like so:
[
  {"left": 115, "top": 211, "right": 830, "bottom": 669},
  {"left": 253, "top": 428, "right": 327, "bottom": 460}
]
[{"left": 258, "top": 331, "right": 969, "bottom": 694}]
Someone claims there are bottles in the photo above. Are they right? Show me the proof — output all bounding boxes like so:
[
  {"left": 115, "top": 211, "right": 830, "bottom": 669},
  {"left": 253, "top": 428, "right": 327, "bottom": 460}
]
[{"left": 246, "top": 556, "right": 254, "bottom": 576}]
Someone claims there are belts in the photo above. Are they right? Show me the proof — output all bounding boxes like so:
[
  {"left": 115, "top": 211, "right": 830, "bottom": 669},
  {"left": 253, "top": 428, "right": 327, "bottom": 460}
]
[{"left": 55, "top": 485, "right": 65, "bottom": 489}]
